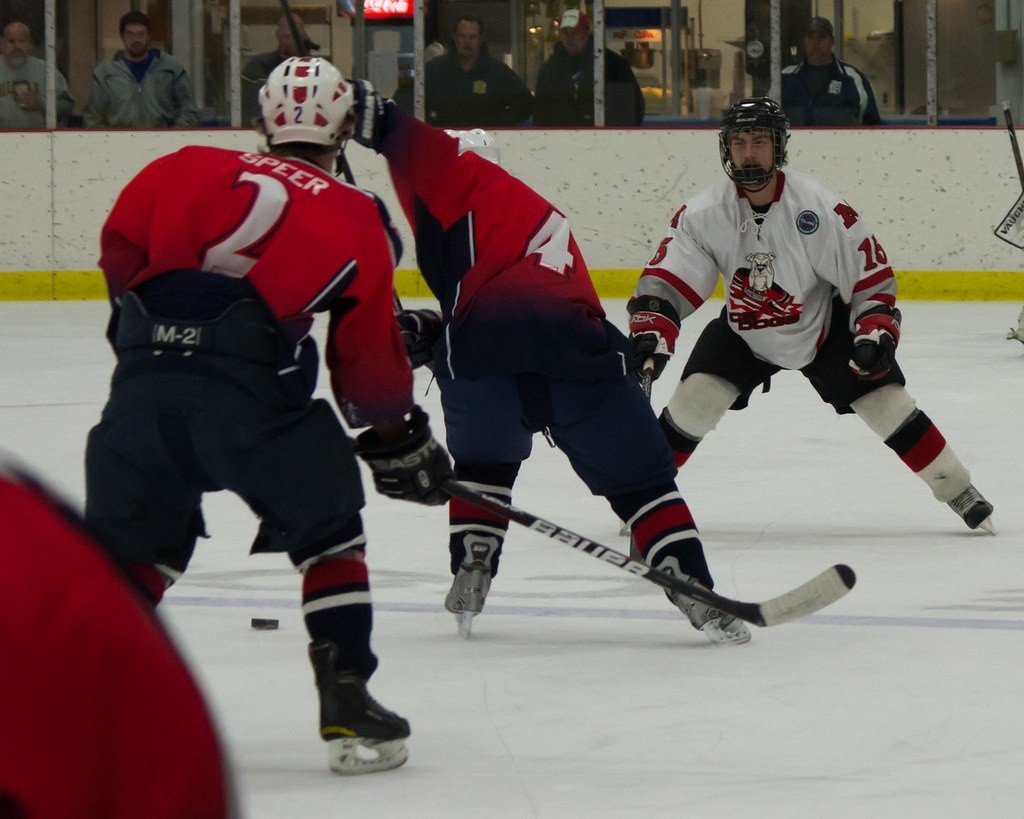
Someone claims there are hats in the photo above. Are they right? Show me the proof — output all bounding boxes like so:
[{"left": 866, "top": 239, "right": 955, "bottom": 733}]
[
  {"left": 559, "top": 9, "right": 590, "bottom": 33},
  {"left": 804, "top": 16, "right": 833, "bottom": 34}
]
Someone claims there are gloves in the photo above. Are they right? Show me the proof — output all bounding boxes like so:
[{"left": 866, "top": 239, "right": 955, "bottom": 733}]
[
  {"left": 626, "top": 296, "right": 683, "bottom": 384},
  {"left": 343, "top": 78, "right": 396, "bottom": 154},
  {"left": 849, "top": 305, "right": 901, "bottom": 382},
  {"left": 394, "top": 307, "right": 454, "bottom": 370},
  {"left": 353, "top": 406, "right": 464, "bottom": 506}
]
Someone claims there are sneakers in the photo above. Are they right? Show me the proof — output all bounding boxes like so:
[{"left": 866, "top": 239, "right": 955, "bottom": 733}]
[
  {"left": 658, "top": 554, "right": 754, "bottom": 648},
  {"left": 443, "top": 531, "right": 502, "bottom": 640},
  {"left": 317, "top": 676, "right": 413, "bottom": 771},
  {"left": 945, "top": 479, "right": 1000, "bottom": 535}
]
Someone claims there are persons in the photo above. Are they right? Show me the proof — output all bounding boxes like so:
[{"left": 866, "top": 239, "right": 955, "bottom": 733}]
[
  {"left": 626, "top": 97, "right": 997, "bottom": 537},
  {"left": 534, "top": 9, "right": 646, "bottom": 127},
  {"left": 241, "top": 14, "right": 307, "bottom": 127},
  {"left": 82, "top": 11, "right": 199, "bottom": 127},
  {"left": 0, "top": 22, "right": 76, "bottom": 130},
  {"left": 83, "top": 57, "right": 455, "bottom": 773},
  {"left": 781, "top": 17, "right": 882, "bottom": 126},
  {"left": 394, "top": 16, "right": 534, "bottom": 125},
  {"left": 345, "top": 78, "right": 755, "bottom": 649},
  {"left": 0, "top": 452, "right": 234, "bottom": 819}
]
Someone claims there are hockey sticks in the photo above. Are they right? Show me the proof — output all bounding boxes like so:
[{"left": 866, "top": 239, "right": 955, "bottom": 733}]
[
  {"left": 629, "top": 356, "right": 657, "bottom": 562},
  {"left": 345, "top": 435, "right": 859, "bottom": 630},
  {"left": 280, "top": 1, "right": 438, "bottom": 376}
]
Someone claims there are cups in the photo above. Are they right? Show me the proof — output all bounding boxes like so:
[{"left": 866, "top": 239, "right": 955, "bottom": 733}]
[{"left": 691, "top": 87, "right": 712, "bottom": 120}]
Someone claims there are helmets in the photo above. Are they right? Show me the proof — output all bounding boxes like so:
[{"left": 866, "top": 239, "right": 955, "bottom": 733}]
[
  {"left": 257, "top": 55, "right": 358, "bottom": 150},
  {"left": 716, "top": 96, "right": 792, "bottom": 190}
]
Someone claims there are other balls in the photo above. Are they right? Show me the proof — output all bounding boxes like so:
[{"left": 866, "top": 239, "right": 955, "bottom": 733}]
[{"left": 250, "top": 618, "right": 279, "bottom": 629}]
